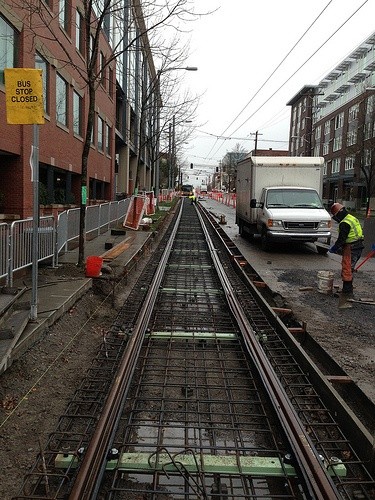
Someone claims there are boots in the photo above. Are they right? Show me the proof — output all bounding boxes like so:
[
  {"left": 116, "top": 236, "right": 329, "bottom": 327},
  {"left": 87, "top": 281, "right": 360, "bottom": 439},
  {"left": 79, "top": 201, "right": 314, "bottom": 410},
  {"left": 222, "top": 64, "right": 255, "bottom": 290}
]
[{"left": 337, "top": 281, "right": 353, "bottom": 294}]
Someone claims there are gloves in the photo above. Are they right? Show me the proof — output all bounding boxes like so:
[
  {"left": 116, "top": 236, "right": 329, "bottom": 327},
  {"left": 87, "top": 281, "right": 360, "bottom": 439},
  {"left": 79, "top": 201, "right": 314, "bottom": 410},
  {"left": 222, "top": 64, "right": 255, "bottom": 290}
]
[{"left": 331, "top": 245, "right": 339, "bottom": 251}]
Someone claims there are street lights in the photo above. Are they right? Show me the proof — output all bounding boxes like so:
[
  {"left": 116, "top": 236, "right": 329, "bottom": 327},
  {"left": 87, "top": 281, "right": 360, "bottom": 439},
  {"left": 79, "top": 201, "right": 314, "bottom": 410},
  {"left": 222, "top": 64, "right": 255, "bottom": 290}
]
[
  {"left": 156, "top": 66, "right": 198, "bottom": 210},
  {"left": 168, "top": 121, "right": 192, "bottom": 188}
]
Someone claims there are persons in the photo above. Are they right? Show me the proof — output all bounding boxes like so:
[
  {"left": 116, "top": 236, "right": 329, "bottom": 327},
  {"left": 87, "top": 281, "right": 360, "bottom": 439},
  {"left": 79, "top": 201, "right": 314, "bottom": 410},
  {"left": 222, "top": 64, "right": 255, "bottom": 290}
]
[{"left": 328, "top": 202, "right": 365, "bottom": 295}]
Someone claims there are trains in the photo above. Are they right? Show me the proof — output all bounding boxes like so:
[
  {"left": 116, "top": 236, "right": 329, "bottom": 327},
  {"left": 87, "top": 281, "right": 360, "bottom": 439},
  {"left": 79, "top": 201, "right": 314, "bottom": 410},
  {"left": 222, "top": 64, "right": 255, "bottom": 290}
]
[{"left": 179, "top": 184, "right": 194, "bottom": 199}]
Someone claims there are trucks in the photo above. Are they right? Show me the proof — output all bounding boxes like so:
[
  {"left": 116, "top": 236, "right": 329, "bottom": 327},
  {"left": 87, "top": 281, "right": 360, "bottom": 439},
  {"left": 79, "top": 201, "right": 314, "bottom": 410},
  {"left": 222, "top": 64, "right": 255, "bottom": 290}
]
[{"left": 235, "top": 156, "right": 332, "bottom": 254}]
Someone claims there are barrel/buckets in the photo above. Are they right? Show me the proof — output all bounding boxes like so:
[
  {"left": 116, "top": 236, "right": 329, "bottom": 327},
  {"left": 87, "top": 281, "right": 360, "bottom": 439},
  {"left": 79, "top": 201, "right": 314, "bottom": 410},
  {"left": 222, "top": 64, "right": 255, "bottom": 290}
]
[
  {"left": 86, "top": 256, "right": 103, "bottom": 277},
  {"left": 317, "top": 271, "right": 334, "bottom": 294}
]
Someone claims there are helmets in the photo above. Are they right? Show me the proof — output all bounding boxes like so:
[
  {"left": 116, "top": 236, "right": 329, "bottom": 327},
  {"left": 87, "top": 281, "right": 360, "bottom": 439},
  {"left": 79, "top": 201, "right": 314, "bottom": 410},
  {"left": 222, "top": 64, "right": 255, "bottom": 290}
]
[{"left": 330, "top": 203, "right": 342, "bottom": 214}]
